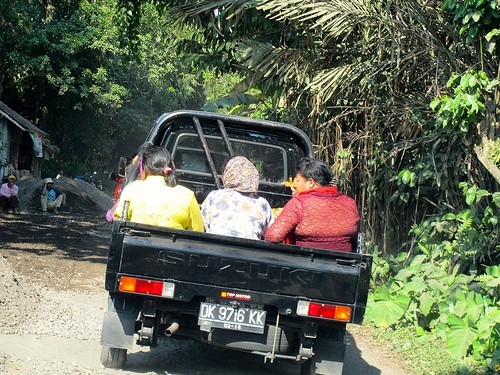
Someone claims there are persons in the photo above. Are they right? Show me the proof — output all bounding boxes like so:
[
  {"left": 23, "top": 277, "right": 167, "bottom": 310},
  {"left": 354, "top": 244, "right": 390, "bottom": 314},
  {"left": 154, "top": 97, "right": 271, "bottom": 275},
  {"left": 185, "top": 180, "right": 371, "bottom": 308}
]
[
  {"left": 40, "top": 177, "right": 67, "bottom": 215},
  {"left": 262, "top": 157, "right": 361, "bottom": 254},
  {"left": 0, "top": 174, "right": 19, "bottom": 214},
  {"left": 113, "top": 145, "right": 206, "bottom": 233},
  {"left": 198, "top": 155, "right": 276, "bottom": 241}
]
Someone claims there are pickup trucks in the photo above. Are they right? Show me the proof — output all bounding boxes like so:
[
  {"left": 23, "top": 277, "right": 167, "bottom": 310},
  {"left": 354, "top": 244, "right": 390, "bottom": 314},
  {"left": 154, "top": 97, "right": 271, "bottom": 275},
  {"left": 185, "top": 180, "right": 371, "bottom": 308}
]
[{"left": 98, "top": 110, "right": 373, "bottom": 375}]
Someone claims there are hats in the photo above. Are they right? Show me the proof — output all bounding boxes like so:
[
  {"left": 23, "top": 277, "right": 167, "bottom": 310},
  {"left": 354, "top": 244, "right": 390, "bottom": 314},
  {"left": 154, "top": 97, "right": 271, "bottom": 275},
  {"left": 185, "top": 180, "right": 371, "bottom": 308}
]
[
  {"left": 46, "top": 177, "right": 54, "bottom": 184},
  {"left": 7, "top": 174, "right": 17, "bottom": 181}
]
